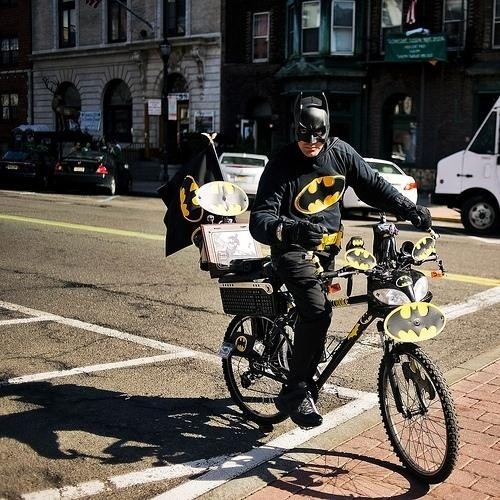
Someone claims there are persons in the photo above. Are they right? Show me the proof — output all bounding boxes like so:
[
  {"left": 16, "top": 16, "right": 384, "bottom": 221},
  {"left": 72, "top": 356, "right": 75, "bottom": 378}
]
[{"left": 249, "top": 91, "right": 433, "bottom": 428}]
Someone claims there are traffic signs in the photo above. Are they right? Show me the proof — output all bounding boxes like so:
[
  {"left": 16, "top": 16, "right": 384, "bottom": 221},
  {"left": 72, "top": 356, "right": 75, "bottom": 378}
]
[{"left": 383, "top": 34, "right": 447, "bottom": 63}]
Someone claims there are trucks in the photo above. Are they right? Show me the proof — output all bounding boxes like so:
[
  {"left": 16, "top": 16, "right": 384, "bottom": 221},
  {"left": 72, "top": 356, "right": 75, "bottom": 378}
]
[{"left": 427, "top": 93, "right": 499, "bottom": 239}]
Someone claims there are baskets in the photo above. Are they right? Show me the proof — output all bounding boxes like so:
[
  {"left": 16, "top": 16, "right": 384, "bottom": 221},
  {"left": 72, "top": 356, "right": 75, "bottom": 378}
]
[{"left": 218, "top": 283, "right": 287, "bottom": 316}]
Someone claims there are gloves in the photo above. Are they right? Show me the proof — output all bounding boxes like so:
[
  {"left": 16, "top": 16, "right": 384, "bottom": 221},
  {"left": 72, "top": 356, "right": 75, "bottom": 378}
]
[
  {"left": 271, "top": 217, "right": 328, "bottom": 248},
  {"left": 390, "top": 194, "right": 432, "bottom": 230}
]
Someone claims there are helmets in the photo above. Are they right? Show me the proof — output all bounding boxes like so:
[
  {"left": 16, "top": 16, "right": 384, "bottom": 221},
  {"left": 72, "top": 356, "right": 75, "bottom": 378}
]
[{"left": 293, "top": 90, "right": 331, "bottom": 143}]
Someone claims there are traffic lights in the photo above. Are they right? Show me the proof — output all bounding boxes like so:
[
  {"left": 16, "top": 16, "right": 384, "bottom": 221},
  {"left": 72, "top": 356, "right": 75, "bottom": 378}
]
[{"left": 85, "top": 0, "right": 103, "bottom": 9}]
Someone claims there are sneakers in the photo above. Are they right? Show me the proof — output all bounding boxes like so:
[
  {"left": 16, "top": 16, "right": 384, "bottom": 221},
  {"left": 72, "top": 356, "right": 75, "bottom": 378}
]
[{"left": 275, "top": 385, "right": 323, "bottom": 428}]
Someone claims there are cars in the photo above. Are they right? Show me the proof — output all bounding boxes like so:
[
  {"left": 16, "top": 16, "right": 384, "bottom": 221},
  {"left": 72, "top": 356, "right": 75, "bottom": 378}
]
[
  {"left": 52, "top": 142, "right": 135, "bottom": 199},
  {"left": 0, "top": 148, "right": 55, "bottom": 191},
  {"left": 340, "top": 156, "right": 419, "bottom": 222},
  {"left": 212, "top": 149, "right": 273, "bottom": 209}
]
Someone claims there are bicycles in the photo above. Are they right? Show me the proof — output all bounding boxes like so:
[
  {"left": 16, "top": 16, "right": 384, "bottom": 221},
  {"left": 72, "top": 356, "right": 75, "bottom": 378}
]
[{"left": 206, "top": 210, "right": 461, "bottom": 484}]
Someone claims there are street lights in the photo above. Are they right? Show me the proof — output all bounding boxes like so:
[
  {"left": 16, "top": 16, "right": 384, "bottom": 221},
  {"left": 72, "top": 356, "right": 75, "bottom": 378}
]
[{"left": 157, "top": 34, "right": 173, "bottom": 186}]
